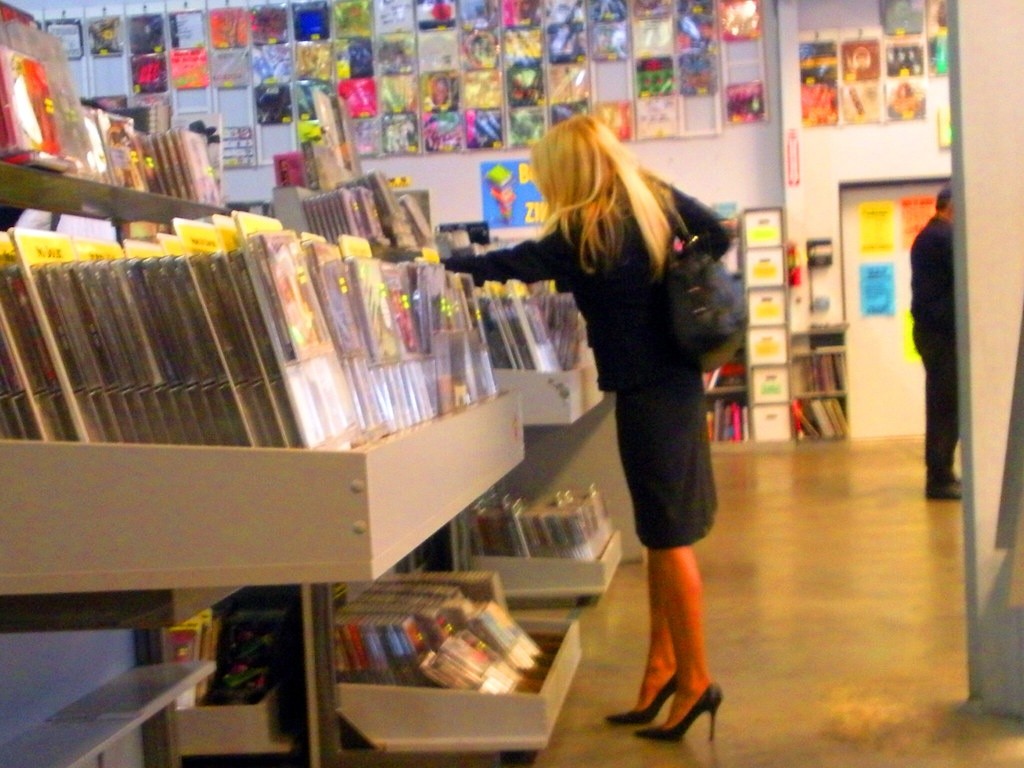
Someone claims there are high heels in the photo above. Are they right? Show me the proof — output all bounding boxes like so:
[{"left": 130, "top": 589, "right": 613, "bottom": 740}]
[
  {"left": 604, "top": 669, "right": 678, "bottom": 725},
  {"left": 634, "top": 681, "right": 724, "bottom": 739}
]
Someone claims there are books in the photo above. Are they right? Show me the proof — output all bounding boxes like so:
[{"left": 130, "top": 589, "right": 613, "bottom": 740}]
[
  {"left": 704, "top": 367, "right": 749, "bottom": 441},
  {"left": 792, "top": 397, "right": 847, "bottom": 440},
  {"left": 807, "top": 351, "right": 845, "bottom": 390}
]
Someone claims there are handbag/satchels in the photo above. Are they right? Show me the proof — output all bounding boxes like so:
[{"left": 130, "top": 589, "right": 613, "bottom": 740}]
[{"left": 662, "top": 200, "right": 750, "bottom": 375}]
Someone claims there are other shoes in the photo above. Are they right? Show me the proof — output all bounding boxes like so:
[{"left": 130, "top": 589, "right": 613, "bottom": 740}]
[{"left": 924, "top": 479, "right": 963, "bottom": 499}]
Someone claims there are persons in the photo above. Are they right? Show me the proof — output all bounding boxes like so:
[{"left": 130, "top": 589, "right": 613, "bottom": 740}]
[
  {"left": 445, "top": 114, "right": 730, "bottom": 741},
  {"left": 911, "top": 186, "right": 962, "bottom": 500}
]
